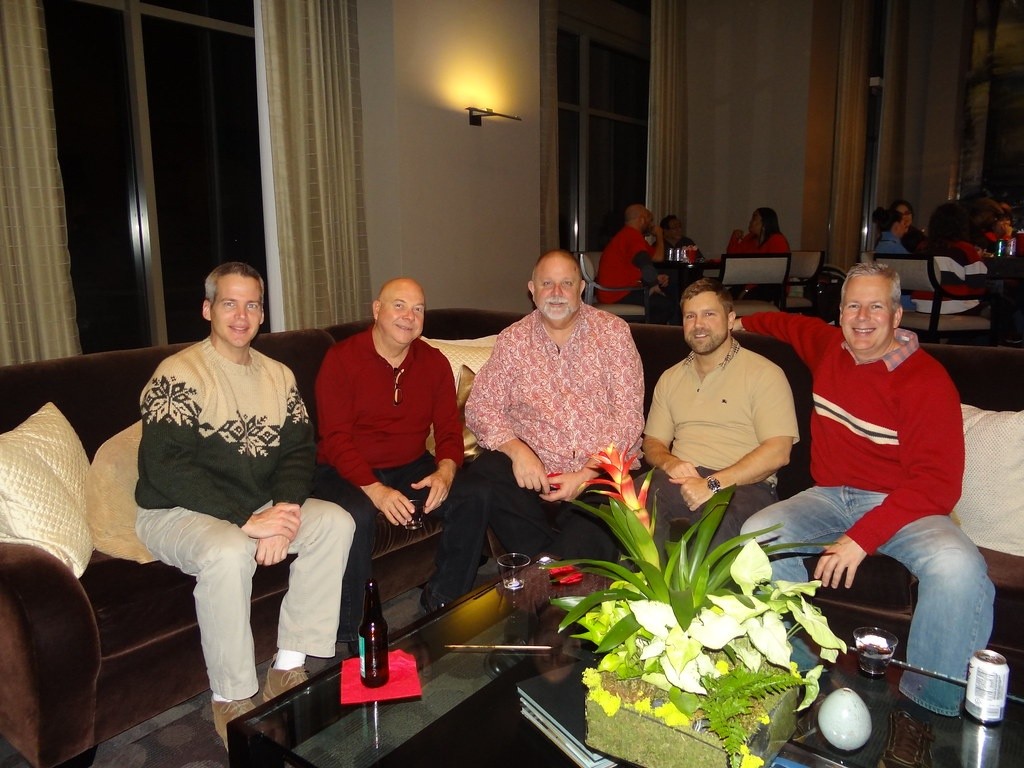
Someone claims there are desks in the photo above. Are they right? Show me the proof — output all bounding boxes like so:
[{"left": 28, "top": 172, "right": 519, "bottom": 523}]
[
  {"left": 656, "top": 261, "right": 722, "bottom": 282},
  {"left": 983, "top": 257, "right": 1024, "bottom": 280}
]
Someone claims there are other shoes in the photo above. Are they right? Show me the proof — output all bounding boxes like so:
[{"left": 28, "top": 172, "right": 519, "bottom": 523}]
[{"left": 420, "top": 582, "right": 448, "bottom": 615}]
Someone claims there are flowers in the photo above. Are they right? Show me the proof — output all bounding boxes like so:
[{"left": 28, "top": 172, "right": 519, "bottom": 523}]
[{"left": 540, "top": 434, "right": 854, "bottom": 768}]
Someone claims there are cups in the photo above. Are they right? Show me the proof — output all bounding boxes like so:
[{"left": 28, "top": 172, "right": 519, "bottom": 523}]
[
  {"left": 496, "top": 552, "right": 531, "bottom": 590},
  {"left": 404, "top": 500, "right": 424, "bottom": 530},
  {"left": 686, "top": 249, "right": 696, "bottom": 264},
  {"left": 1016, "top": 233, "right": 1024, "bottom": 256},
  {"left": 852, "top": 626, "right": 898, "bottom": 679}
]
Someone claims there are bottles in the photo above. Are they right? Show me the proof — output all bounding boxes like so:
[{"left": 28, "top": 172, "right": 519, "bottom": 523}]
[{"left": 358, "top": 578, "right": 389, "bottom": 686}]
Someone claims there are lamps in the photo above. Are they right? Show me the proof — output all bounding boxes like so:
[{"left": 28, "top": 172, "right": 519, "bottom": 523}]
[{"left": 466, "top": 106, "right": 522, "bottom": 126}]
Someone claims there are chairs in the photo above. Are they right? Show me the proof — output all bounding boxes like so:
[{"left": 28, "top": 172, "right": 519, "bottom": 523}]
[
  {"left": 873, "top": 252, "right": 993, "bottom": 345},
  {"left": 576, "top": 251, "right": 649, "bottom": 322},
  {"left": 718, "top": 252, "right": 793, "bottom": 317},
  {"left": 786, "top": 249, "right": 825, "bottom": 312}
]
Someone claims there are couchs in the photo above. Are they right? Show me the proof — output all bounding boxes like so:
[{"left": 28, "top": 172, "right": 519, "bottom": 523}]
[{"left": 0, "top": 306, "right": 1024, "bottom": 768}]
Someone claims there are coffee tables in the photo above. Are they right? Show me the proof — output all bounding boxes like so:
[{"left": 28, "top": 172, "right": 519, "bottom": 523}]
[{"left": 226, "top": 551, "right": 1024, "bottom": 768}]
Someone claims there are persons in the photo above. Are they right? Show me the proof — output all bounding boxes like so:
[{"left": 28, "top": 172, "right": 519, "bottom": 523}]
[
  {"left": 133, "top": 262, "right": 357, "bottom": 755},
  {"left": 594, "top": 204, "right": 706, "bottom": 325},
  {"left": 726, "top": 208, "right": 791, "bottom": 302},
  {"left": 639, "top": 277, "right": 799, "bottom": 584},
  {"left": 312, "top": 277, "right": 492, "bottom": 660},
  {"left": 465, "top": 246, "right": 647, "bottom": 681},
  {"left": 728, "top": 263, "right": 997, "bottom": 767},
  {"left": 871, "top": 194, "right": 1024, "bottom": 344}
]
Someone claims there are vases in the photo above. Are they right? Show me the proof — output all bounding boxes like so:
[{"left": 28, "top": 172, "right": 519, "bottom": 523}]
[{"left": 583, "top": 669, "right": 798, "bottom": 768}]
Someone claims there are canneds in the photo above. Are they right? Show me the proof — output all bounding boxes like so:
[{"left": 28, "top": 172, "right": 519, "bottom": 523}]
[
  {"left": 995, "top": 239, "right": 1004, "bottom": 257},
  {"left": 964, "top": 649, "right": 1009, "bottom": 723},
  {"left": 1007, "top": 239, "right": 1016, "bottom": 255},
  {"left": 667, "top": 247, "right": 681, "bottom": 261}
]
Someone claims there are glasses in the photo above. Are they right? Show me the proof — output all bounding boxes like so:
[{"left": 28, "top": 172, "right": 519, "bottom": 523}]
[{"left": 900, "top": 210, "right": 912, "bottom": 215}]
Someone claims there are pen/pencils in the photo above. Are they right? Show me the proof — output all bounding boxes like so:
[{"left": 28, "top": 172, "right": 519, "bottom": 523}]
[{"left": 444, "top": 645, "right": 554, "bottom": 650}]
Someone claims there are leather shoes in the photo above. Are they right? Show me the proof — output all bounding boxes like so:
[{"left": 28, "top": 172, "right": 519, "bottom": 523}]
[{"left": 484, "top": 630, "right": 530, "bottom": 680}]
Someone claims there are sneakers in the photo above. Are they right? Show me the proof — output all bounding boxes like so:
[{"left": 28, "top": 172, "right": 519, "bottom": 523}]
[
  {"left": 792, "top": 683, "right": 871, "bottom": 741},
  {"left": 877, "top": 693, "right": 936, "bottom": 768},
  {"left": 211, "top": 695, "right": 286, "bottom": 755},
  {"left": 263, "top": 652, "right": 343, "bottom": 725}
]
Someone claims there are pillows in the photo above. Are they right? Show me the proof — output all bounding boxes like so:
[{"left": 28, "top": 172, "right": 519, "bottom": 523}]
[
  {"left": 424, "top": 364, "right": 482, "bottom": 458},
  {"left": 0, "top": 397, "right": 96, "bottom": 579},
  {"left": 425, "top": 332, "right": 500, "bottom": 384},
  {"left": 86, "top": 422, "right": 159, "bottom": 565},
  {"left": 949, "top": 404, "right": 1024, "bottom": 554}
]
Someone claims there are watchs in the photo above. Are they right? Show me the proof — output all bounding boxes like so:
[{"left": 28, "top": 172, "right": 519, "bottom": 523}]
[{"left": 707, "top": 476, "right": 721, "bottom": 493}]
